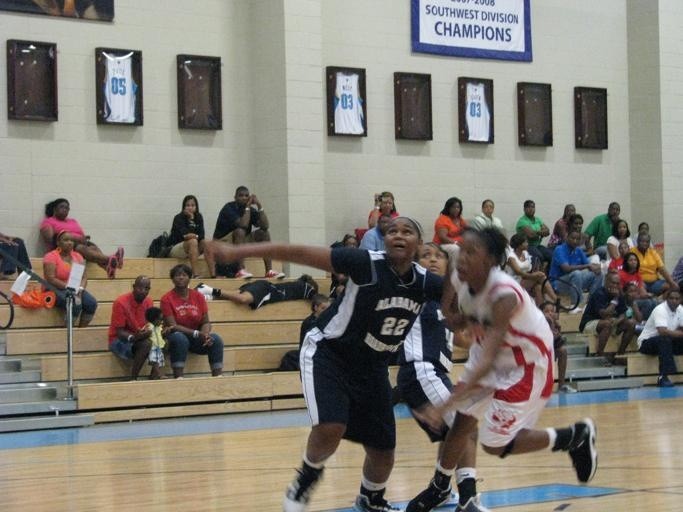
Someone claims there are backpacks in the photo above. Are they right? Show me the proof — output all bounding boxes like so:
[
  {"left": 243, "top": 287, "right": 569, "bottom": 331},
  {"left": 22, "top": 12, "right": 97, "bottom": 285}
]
[{"left": 263, "top": 349, "right": 301, "bottom": 373}]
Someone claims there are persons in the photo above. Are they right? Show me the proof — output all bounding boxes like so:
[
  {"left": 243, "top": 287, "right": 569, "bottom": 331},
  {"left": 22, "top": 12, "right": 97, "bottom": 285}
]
[
  {"left": 366, "top": 190, "right": 399, "bottom": 226},
  {"left": 107, "top": 273, "right": 161, "bottom": 382},
  {"left": 0, "top": 231, "right": 34, "bottom": 281},
  {"left": 356, "top": 214, "right": 395, "bottom": 255},
  {"left": 211, "top": 186, "right": 286, "bottom": 281},
  {"left": 164, "top": 195, "right": 226, "bottom": 279},
  {"left": 138, "top": 306, "right": 169, "bottom": 380},
  {"left": 418, "top": 222, "right": 602, "bottom": 483},
  {"left": 328, "top": 233, "right": 360, "bottom": 302},
  {"left": 159, "top": 263, "right": 226, "bottom": 379},
  {"left": 430, "top": 195, "right": 470, "bottom": 251},
  {"left": 39, "top": 197, "right": 124, "bottom": 280},
  {"left": 392, "top": 238, "right": 493, "bottom": 511},
  {"left": 471, "top": 198, "right": 504, "bottom": 234},
  {"left": 42, "top": 228, "right": 96, "bottom": 327},
  {"left": 297, "top": 294, "right": 332, "bottom": 349},
  {"left": 192, "top": 271, "right": 320, "bottom": 310},
  {"left": 501, "top": 198, "right": 682, "bottom": 395},
  {"left": 197, "top": 214, "right": 475, "bottom": 511}
]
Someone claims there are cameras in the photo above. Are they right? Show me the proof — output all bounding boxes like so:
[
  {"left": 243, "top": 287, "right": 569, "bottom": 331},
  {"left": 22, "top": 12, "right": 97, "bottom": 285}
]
[{"left": 377, "top": 195, "right": 388, "bottom": 203}]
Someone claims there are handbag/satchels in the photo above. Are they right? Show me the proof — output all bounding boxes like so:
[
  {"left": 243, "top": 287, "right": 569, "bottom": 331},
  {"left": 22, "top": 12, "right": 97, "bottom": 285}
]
[
  {"left": 146, "top": 231, "right": 172, "bottom": 258},
  {"left": 10, "top": 284, "right": 57, "bottom": 310}
]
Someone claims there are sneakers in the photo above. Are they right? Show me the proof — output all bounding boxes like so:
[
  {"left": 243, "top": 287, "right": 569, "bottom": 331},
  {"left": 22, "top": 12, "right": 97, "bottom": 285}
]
[
  {"left": 263, "top": 269, "right": 286, "bottom": 280},
  {"left": 354, "top": 491, "right": 404, "bottom": 511},
  {"left": 595, "top": 356, "right": 613, "bottom": 367},
  {"left": 233, "top": 270, "right": 254, "bottom": 280},
  {"left": 562, "top": 304, "right": 584, "bottom": 314},
  {"left": 656, "top": 373, "right": 675, "bottom": 388},
  {"left": 454, "top": 491, "right": 491, "bottom": 512},
  {"left": 280, "top": 461, "right": 327, "bottom": 512},
  {"left": 104, "top": 255, "right": 118, "bottom": 278},
  {"left": 1, "top": 273, "right": 18, "bottom": 281},
  {"left": 556, "top": 384, "right": 578, "bottom": 393},
  {"left": 563, "top": 416, "right": 600, "bottom": 485},
  {"left": 404, "top": 472, "right": 461, "bottom": 512},
  {"left": 112, "top": 245, "right": 125, "bottom": 270},
  {"left": 192, "top": 282, "right": 213, "bottom": 300}
]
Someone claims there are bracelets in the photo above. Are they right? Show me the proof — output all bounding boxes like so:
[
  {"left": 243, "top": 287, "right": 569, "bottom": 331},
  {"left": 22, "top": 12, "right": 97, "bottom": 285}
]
[
  {"left": 454, "top": 240, "right": 457, "bottom": 244},
  {"left": 77, "top": 286, "right": 84, "bottom": 292},
  {"left": 257, "top": 208, "right": 263, "bottom": 212},
  {"left": 373, "top": 206, "right": 380, "bottom": 210},
  {"left": 244, "top": 207, "right": 250, "bottom": 211},
  {"left": 127, "top": 335, "right": 133, "bottom": 341},
  {"left": 192, "top": 328, "right": 199, "bottom": 339},
  {"left": 195, "top": 234, "right": 199, "bottom": 239}
]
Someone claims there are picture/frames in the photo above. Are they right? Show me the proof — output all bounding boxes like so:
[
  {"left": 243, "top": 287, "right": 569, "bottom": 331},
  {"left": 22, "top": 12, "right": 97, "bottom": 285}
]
[
  {"left": 392, "top": 70, "right": 432, "bottom": 140},
  {"left": 176, "top": 54, "right": 223, "bottom": 130},
  {"left": 93, "top": 46, "right": 144, "bottom": 128},
  {"left": 457, "top": 76, "right": 494, "bottom": 144},
  {"left": 7, "top": 37, "right": 59, "bottom": 123},
  {"left": 573, "top": 85, "right": 608, "bottom": 149},
  {"left": 517, "top": 81, "right": 552, "bottom": 147},
  {"left": 325, "top": 65, "right": 367, "bottom": 137}
]
